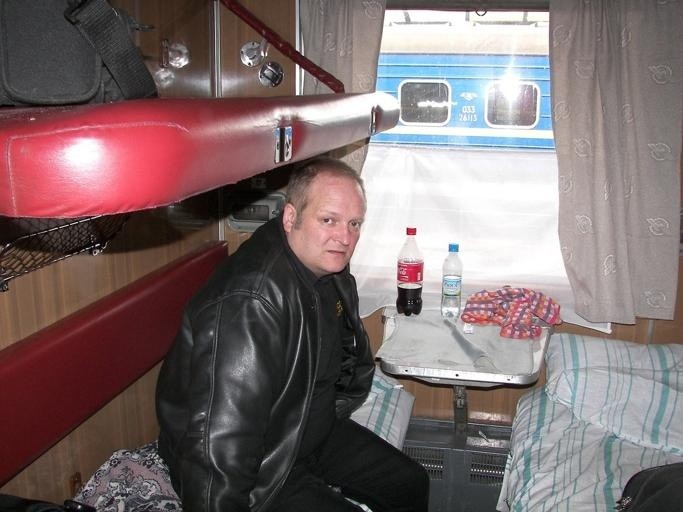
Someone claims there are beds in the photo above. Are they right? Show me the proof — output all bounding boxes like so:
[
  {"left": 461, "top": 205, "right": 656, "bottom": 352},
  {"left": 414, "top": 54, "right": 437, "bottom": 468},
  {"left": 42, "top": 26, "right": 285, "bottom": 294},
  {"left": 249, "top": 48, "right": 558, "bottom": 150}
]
[
  {"left": 75, "top": 366, "right": 418, "bottom": 512},
  {"left": 495, "top": 386, "right": 680, "bottom": 512}
]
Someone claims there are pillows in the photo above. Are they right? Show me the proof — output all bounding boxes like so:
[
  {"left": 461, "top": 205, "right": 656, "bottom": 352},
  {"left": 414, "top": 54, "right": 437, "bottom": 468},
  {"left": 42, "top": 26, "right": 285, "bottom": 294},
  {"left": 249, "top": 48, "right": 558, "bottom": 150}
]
[
  {"left": 363, "top": 364, "right": 399, "bottom": 407},
  {"left": 543, "top": 331, "right": 683, "bottom": 404}
]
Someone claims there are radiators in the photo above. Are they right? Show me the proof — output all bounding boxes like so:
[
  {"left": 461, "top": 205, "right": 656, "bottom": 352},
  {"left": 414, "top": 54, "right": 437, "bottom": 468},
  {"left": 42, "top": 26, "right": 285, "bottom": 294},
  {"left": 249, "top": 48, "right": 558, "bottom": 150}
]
[{"left": 400, "top": 424, "right": 513, "bottom": 511}]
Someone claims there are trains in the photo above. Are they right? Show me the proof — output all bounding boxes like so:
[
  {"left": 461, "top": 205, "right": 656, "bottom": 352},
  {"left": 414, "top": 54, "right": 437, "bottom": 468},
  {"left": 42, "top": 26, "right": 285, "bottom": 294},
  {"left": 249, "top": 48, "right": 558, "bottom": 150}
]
[{"left": 356, "top": 23, "right": 609, "bottom": 328}]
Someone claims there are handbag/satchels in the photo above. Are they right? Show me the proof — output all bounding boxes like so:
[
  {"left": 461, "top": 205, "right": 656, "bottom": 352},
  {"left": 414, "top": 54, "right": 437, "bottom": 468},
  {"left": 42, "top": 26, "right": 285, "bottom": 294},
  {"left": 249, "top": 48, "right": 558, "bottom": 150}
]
[{"left": 0, "top": 0, "right": 159, "bottom": 107}]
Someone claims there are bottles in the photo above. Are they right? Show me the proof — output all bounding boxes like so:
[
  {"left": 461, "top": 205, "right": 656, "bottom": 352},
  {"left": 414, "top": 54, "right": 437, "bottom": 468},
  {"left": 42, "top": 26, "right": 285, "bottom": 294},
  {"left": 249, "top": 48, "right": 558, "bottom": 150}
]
[
  {"left": 440, "top": 243, "right": 463, "bottom": 321},
  {"left": 395, "top": 226, "right": 424, "bottom": 315}
]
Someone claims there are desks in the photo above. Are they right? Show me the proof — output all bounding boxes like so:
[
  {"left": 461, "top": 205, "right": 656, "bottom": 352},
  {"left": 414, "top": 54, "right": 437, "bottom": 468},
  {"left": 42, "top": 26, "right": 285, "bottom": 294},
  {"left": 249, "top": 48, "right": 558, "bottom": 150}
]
[{"left": 378, "top": 315, "right": 551, "bottom": 443}]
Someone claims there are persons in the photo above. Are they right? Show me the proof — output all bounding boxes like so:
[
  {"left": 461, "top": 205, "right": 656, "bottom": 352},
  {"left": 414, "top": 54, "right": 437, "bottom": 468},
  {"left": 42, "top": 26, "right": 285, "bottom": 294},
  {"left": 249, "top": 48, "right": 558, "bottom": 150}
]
[{"left": 156, "top": 160, "right": 429, "bottom": 512}]
